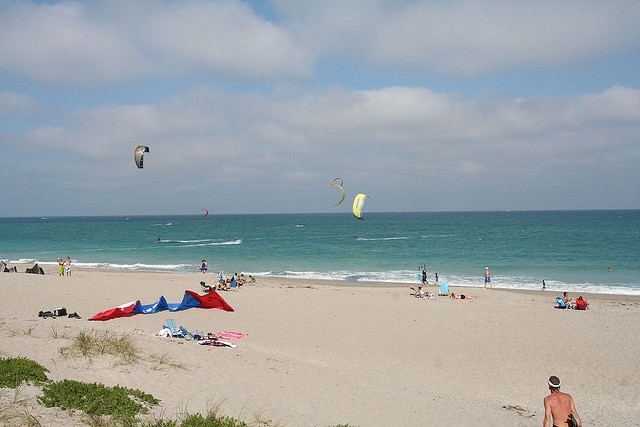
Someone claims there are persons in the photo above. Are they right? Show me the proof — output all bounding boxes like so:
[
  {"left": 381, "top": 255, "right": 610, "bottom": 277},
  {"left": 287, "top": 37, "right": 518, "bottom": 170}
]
[
  {"left": 450, "top": 293, "right": 473, "bottom": 299},
  {"left": 562, "top": 291, "right": 587, "bottom": 310},
  {"left": 543, "top": 375, "right": 582, "bottom": 427},
  {"left": 410, "top": 287, "right": 433, "bottom": 298},
  {"left": 542, "top": 280, "right": 546, "bottom": 289},
  {"left": 57, "top": 258, "right": 64, "bottom": 276},
  {"left": 64, "top": 257, "right": 71, "bottom": 277},
  {"left": 419, "top": 267, "right": 428, "bottom": 285},
  {"left": 200, "top": 259, "right": 207, "bottom": 273},
  {"left": 200, "top": 272, "right": 256, "bottom": 294},
  {"left": 434, "top": 273, "right": 438, "bottom": 284},
  {"left": 483, "top": 267, "right": 492, "bottom": 289}
]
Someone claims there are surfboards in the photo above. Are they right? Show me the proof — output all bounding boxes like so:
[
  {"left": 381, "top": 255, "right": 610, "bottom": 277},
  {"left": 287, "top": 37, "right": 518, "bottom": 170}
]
[{"left": 571, "top": 413, "right": 578, "bottom": 427}]
